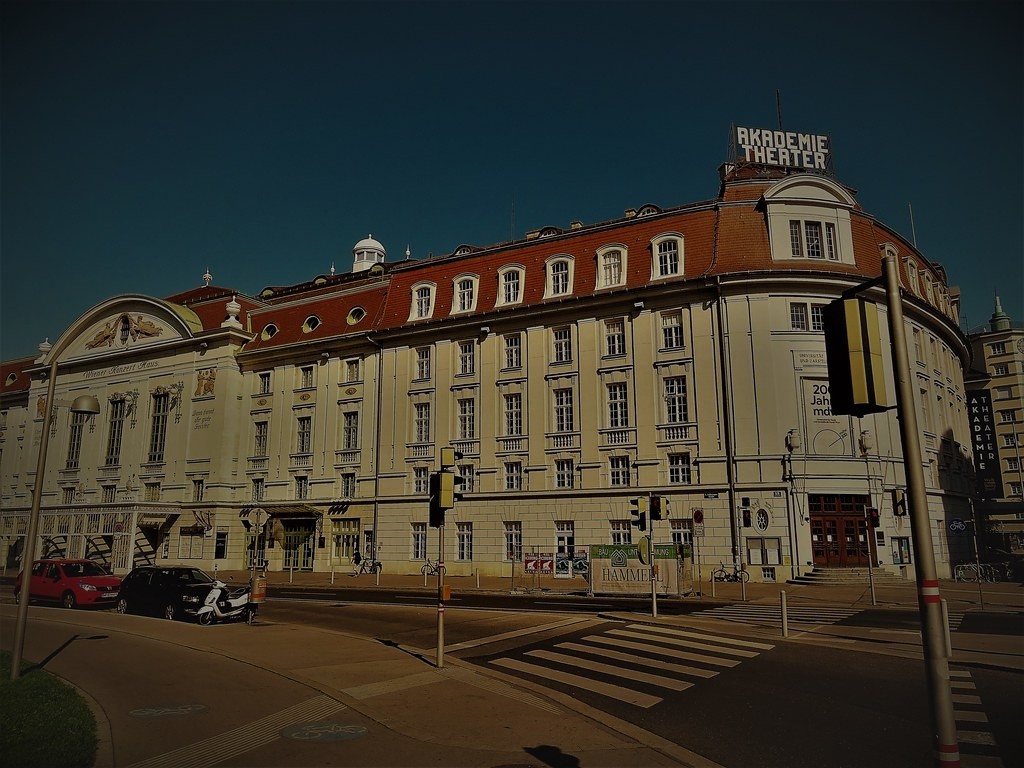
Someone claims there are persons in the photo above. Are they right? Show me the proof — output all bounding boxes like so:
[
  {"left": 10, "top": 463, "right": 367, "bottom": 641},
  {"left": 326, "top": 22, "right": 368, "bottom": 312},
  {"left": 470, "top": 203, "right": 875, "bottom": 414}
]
[
  {"left": 85, "top": 322, "right": 116, "bottom": 348},
  {"left": 194, "top": 368, "right": 216, "bottom": 397},
  {"left": 131, "top": 315, "right": 163, "bottom": 335},
  {"left": 351, "top": 548, "right": 361, "bottom": 578},
  {"left": 37, "top": 395, "right": 45, "bottom": 418}
]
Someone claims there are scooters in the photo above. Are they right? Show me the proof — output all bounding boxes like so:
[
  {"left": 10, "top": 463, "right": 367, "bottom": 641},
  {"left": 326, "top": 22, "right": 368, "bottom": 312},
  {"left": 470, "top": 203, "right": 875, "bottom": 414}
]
[{"left": 197, "top": 575, "right": 258, "bottom": 626}]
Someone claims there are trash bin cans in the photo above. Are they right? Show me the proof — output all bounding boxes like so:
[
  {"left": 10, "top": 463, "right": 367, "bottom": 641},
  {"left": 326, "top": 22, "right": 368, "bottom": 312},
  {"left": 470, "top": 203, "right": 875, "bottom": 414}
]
[{"left": 251, "top": 574, "right": 267, "bottom": 603}]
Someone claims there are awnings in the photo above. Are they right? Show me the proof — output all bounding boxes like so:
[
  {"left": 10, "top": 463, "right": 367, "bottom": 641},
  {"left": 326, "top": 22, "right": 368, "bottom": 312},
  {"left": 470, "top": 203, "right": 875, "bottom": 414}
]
[{"left": 229, "top": 503, "right": 324, "bottom": 516}]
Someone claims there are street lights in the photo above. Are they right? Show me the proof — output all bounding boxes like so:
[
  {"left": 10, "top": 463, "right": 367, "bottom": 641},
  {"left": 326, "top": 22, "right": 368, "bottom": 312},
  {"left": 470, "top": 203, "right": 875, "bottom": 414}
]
[{"left": 8, "top": 361, "right": 101, "bottom": 681}]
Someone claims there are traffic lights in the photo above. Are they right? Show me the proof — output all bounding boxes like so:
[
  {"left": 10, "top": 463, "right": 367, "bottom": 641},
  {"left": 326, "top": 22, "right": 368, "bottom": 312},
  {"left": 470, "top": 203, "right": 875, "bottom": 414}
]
[
  {"left": 440, "top": 471, "right": 464, "bottom": 510},
  {"left": 870, "top": 508, "right": 879, "bottom": 528},
  {"left": 440, "top": 447, "right": 465, "bottom": 468},
  {"left": 650, "top": 495, "right": 670, "bottom": 522},
  {"left": 630, "top": 497, "right": 648, "bottom": 532}
]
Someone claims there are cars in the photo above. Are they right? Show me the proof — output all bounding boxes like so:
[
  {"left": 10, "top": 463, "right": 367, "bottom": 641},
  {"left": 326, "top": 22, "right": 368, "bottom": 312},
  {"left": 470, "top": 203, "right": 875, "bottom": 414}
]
[
  {"left": 14, "top": 559, "right": 122, "bottom": 611},
  {"left": 115, "top": 565, "right": 232, "bottom": 621}
]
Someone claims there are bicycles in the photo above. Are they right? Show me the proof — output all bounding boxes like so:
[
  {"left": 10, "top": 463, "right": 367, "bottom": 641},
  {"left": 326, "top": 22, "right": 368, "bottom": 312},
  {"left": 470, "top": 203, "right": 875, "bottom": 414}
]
[
  {"left": 713, "top": 559, "right": 749, "bottom": 584},
  {"left": 359, "top": 555, "right": 383, "bottom": 574},
  {"left": 958, "top": 561, "right": 1002, "bottom": 585},
  {"left": 420, "top": 558, "right": 447, "bottom": 577}
]
